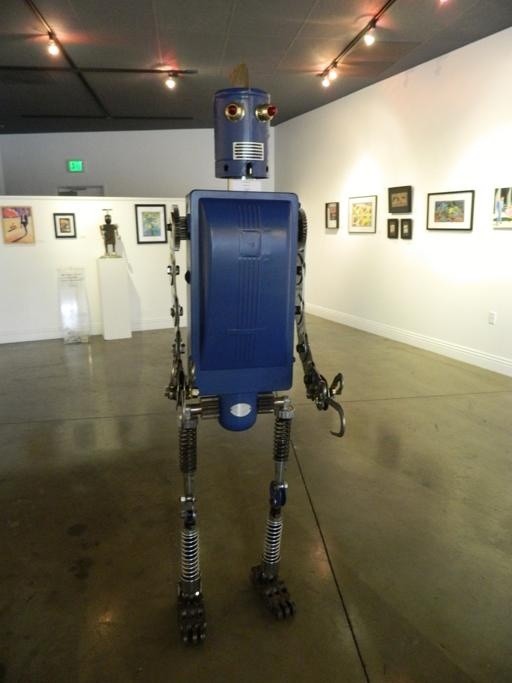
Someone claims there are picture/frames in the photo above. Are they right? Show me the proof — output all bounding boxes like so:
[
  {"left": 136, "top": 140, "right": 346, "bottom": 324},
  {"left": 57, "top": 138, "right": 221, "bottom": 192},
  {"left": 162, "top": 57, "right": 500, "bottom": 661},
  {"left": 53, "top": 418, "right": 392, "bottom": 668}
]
[
  {"left": 54, "top": 213, "right": 76, "bottom": 238},
  {"left": 427, "top": 190, "right": 474, "bottom": 230},
  {"left": 349, "top": 195, "right": 377, "bottom": 233},
  {"left": 135, "top": 204, "right": 167, "bottom": 243},
  {"left": 326, "top": 202, "right": 338, "bottom": 229},
  {"left": 388, "top": 185, "right": 412, "bottom": 239}
]
